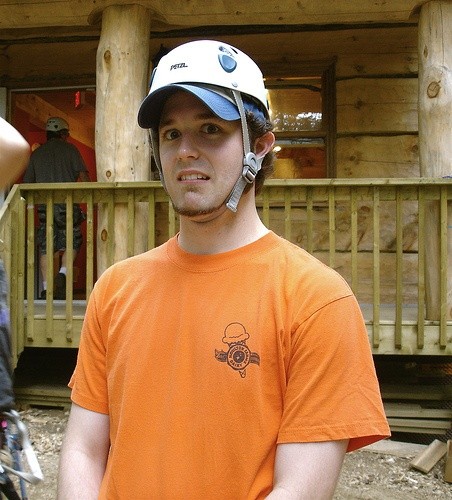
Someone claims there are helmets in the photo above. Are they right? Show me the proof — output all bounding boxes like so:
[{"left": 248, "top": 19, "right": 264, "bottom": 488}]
[
  {"left": 147, "top": 38, "right": 275, "bottom": 122},
  {"left": 44, "top": 117, "right": 71, "bottom": 133}
]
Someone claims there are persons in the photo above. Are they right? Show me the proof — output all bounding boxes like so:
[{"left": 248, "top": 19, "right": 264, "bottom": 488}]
[
  {"left": 0, "top": 117, "right": 43, "bottom": 500},
  {"left": 21, "top": 117, "right": 90, "bottom": 300},
  {"left": 58, "top": 41, "right": 392, "bottom": 500}
]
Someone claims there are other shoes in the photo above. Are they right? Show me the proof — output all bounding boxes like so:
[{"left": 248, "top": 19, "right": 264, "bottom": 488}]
[
  {"left": 41, "top": 289, "right": 47, "bottom": 299},
  {"left": 55, "top": 273, "right": 67, "bottom": 285}
]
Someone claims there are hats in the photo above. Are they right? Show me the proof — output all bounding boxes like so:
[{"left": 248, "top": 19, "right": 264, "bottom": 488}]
[{"left": 135, "top": 83, "right": 270, "bottom": 127}]
